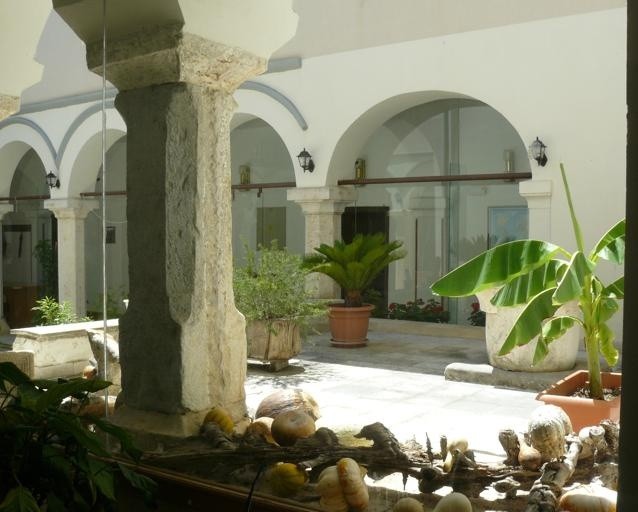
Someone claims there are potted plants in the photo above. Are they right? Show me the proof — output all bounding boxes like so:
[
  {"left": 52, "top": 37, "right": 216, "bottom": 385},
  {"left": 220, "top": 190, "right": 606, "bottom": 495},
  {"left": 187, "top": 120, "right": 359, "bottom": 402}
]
[
  {"left": 231, "top": 228, "right": 407, "bottom": 374},
  {"left": 427, "top": 163, "right": 629, "bottom": 432}
]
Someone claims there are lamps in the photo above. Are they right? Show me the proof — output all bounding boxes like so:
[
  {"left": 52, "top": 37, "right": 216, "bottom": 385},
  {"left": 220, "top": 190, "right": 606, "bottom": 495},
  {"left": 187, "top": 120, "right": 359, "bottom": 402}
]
[
  {"left": 531, "top": 136, "right": 548, "bottom": 168},
  {"left": 46, "top": 170, "right": 60, "bottom": 189},
  {"left": 296, "top": 147, "right": 315, "bottom": 174}
]
